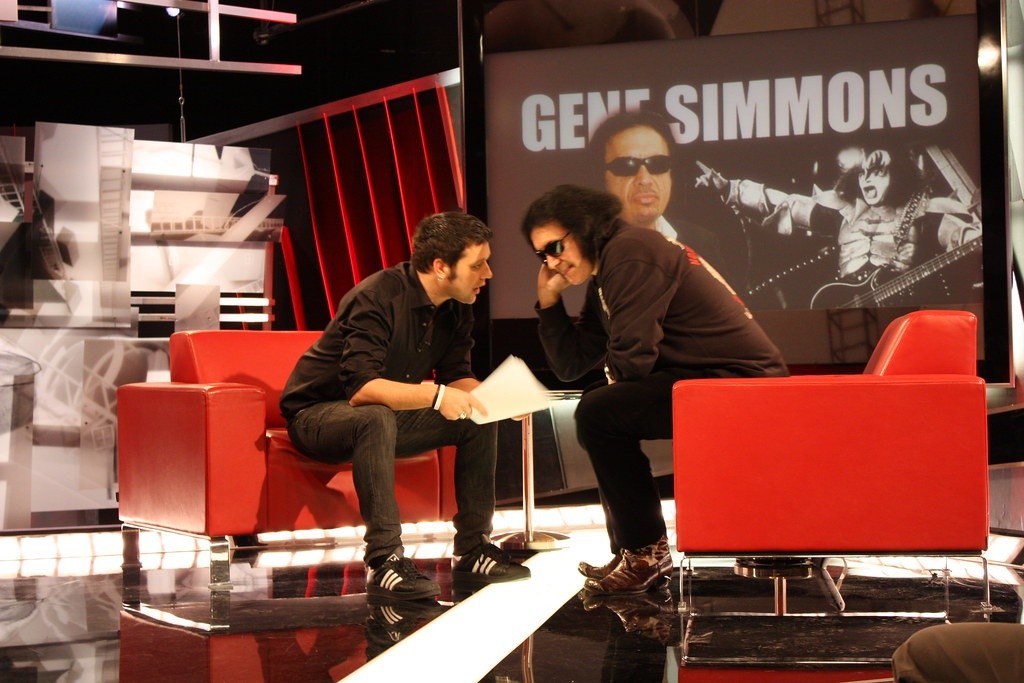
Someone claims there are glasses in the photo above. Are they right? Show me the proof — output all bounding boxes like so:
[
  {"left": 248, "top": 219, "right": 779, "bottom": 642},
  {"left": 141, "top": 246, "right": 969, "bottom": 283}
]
[
  {"left": 535, "top": 230, "right": 572, "bottom": 266},
  {"left": 604, "top": 155, "right": 672, "bottom": 177}
]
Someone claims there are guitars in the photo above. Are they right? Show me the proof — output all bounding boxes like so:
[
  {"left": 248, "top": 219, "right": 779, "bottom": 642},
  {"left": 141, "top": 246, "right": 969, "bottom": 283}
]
[{"left": 810, "top": 234, "right": 983, "bottom": 308}]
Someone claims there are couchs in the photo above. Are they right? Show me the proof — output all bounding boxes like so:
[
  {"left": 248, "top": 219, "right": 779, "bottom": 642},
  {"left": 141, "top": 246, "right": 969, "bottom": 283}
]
[
  {"left": 114, "top": 329, "right": 458, "bottom": 583},
  {"left": 673, "top": 307, "right": 996, "bottom": 616}
]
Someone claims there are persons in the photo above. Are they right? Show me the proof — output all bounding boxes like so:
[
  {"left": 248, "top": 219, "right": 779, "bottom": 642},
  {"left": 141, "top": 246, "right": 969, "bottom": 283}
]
[
  {"left": 892, "top": 623, "right": 1024, "bottom": 683},
  {"left": 361, "top": 596, "right": 686, "bottom": 683},
  {"left": 279, "top": 213, "right": 531, "bottom": 597},
  {"left": 579, "top": 112, "right": 730, "bottom": 285},
  {"left": 690, "top": 144, "right": 982, "bottom": 307},
  {"left": 523, "top": 185, "right": 787, "bottom": 595}
]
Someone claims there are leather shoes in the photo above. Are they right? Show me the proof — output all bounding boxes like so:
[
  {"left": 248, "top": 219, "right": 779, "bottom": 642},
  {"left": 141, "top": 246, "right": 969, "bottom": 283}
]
[
  {"left": 583, "top": 588, "right": 674, "bottom": 638},
  {"left": 584, "top": 555, "right": 675, "bottom": 595},
  {"left": 577, "top": 556, "right": 625, "bottom": 579}
]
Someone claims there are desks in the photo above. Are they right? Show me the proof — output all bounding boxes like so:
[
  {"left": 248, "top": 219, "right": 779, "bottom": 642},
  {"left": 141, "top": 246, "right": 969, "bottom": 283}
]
[{"left": 473, "top": 387, "right": 605, "bottom": 550}]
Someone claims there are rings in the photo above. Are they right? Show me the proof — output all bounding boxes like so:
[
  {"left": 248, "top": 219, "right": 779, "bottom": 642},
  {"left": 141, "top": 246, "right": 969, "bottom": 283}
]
[{"left": 460, "top": 411, "right": 466, "bottom": 419}]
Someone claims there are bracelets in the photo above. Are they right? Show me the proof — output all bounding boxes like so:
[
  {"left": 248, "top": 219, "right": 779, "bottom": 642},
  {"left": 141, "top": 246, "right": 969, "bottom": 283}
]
[{"left": 432, "top": 384, "right": 445, "bottom": 410}]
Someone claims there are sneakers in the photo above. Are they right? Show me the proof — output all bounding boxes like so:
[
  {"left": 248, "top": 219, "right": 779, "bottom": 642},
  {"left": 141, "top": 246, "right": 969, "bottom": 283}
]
[
  {"left": 366, "top": 557, "right": 441, "bottom": 598},
  {"left": 451, "top": 541, "right": 532, "bottom": 583},
  {"left": 450, "top": 589, "right": 476, "bottom": 606},
  {"left": 367, "top": 598, "right": 443, "bottom": 642}
]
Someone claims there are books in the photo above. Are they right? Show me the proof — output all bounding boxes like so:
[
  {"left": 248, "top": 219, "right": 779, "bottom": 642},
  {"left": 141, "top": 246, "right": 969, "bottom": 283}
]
[{"left": 468, "top": 357, "right": 549, "bottom": 424}]
